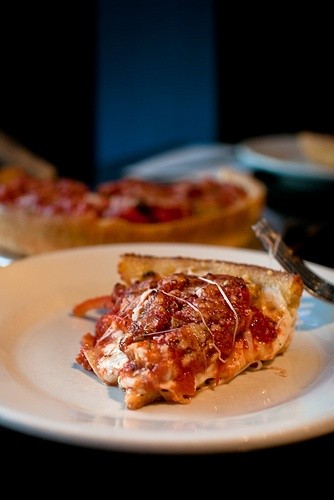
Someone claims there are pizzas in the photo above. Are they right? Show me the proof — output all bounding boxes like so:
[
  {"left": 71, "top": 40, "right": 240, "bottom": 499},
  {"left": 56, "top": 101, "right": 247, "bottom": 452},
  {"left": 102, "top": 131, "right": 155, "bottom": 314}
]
[
  {"left": 0, "top": 168, "right": 268, "bottom": 254},
  {"left": 75, "top": 252, "right": 305, "bottom": 412}
]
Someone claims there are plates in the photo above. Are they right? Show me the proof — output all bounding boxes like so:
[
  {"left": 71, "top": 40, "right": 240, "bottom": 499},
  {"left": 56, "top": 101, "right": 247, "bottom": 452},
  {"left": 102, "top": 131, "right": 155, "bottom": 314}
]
[{"left": 1, "top": 240, "right": 332, "bottom": 455}]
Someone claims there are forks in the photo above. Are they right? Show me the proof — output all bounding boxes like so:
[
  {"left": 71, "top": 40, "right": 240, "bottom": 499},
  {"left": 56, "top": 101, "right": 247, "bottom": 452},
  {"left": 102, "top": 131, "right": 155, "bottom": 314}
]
[{"left": 250, "top": 217, "right": 334, "bottom": 300}]
